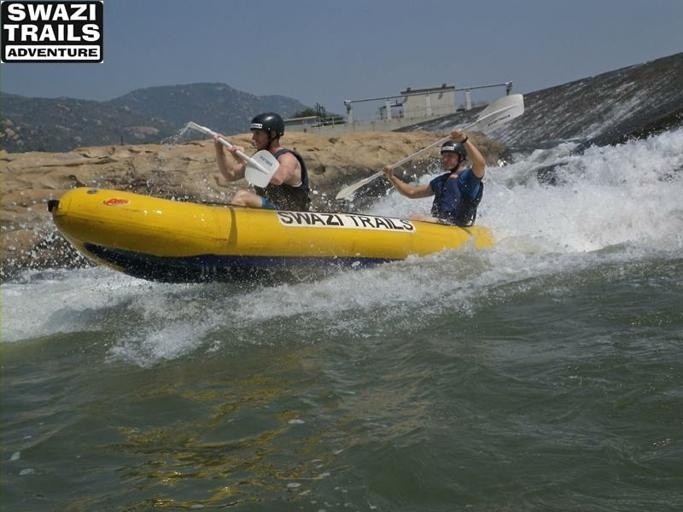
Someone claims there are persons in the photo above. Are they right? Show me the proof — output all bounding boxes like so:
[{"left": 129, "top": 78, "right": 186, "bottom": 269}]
[
  {"left": 379, "top": 129, "right": 486, "bottom": 230},
  {"left": 213, "top": 111, "right": 311, "bottom": 213}
]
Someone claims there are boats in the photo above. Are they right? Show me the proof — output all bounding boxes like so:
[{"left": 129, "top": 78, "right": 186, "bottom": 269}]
[{"left": 48, "top": 187, "right": 498, "bottom": 283}]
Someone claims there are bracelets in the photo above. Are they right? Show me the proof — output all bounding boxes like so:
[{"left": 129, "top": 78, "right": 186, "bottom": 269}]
[{"left": 460, "top": 136, "right": 468, "bottom": 144}]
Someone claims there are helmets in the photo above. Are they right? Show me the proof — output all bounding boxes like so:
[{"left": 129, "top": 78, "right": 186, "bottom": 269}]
[
  {"left": 248, "top": 112, "right": 285, "bottom": 146},
  {"left": 440, "top": 140, "right": 468, "bottom": 165}
]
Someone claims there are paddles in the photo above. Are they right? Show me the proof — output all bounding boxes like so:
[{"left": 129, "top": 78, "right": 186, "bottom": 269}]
[
  {"left": 187, "top": 121, "right": 280, "bottom": 188},
  {"left": 335, "top": 93, "right": 524, "bottom": 200}
]
